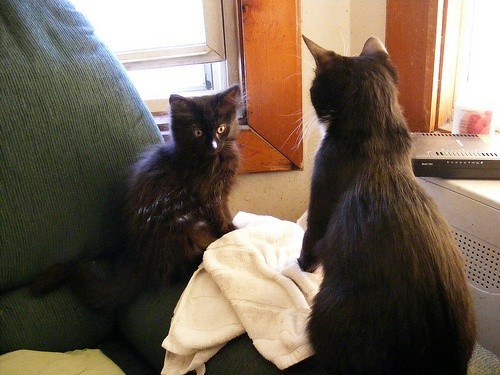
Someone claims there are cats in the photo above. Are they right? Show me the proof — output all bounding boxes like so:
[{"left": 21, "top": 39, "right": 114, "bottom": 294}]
[
  {"left": 296, "top": 33, "right": 478, "bottom": 375},
  {"left": 24, "top": 85, "right": 250, "bottom": 316}
]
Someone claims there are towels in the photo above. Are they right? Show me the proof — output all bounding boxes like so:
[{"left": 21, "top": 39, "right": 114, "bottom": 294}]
[{"left": 157, "top": 209, "right": 325, "bottom": 375}]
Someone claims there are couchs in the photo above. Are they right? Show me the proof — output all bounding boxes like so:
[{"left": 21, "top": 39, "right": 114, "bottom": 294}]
[{"left": 0, "top": 0, "right": 500, "bottom": 375}]
